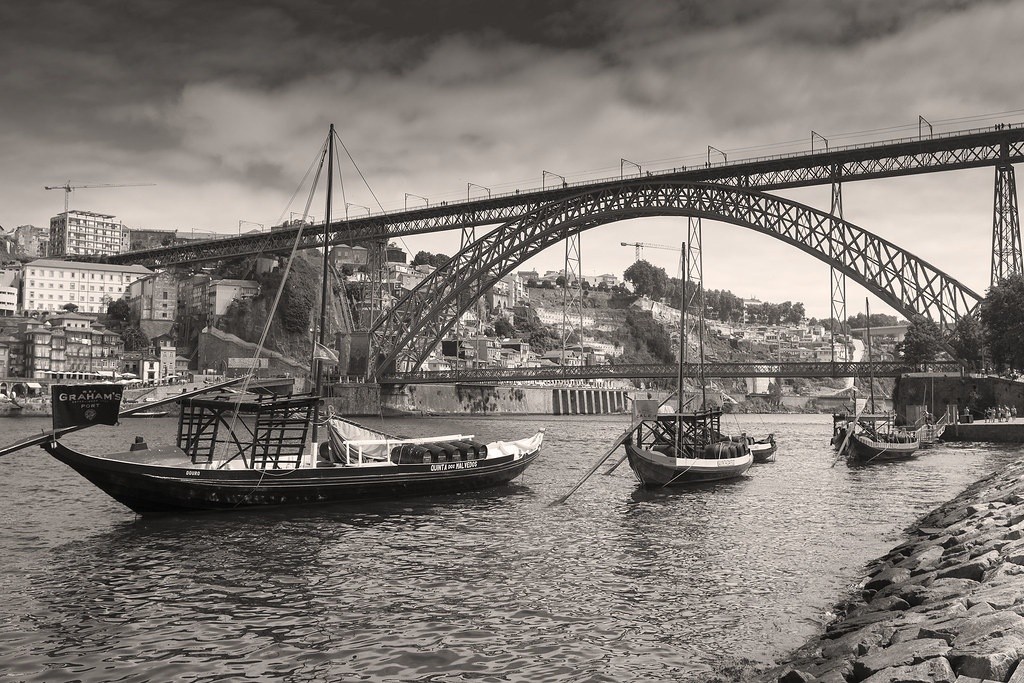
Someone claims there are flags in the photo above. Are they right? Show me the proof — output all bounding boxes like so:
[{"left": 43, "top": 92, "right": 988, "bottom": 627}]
[
  {"left": 52, "top": 385, "right": 124, "bottom": 426},
  {"left": 314, "top": 342, "right": 339, "bottom": 367}
]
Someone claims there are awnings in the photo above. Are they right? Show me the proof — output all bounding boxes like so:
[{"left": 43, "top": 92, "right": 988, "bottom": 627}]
[{"left": 26, "top": 382, "right": 42, "bottom": 392}]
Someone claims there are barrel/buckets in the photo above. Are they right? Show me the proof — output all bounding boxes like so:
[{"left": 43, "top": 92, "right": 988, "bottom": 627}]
[
  {"left": 705, "top": 445, "right": 732, "bottom": 459},
  {"left": 391, "top": 446, "right": 434, "bottom": 463},
  {"left": 728, "top": 441, "right": 746, "bottom": 456},
  {"left": 462, "top": 440, "right": 488, "bottom": 460},
  {"left": 419, "top": 444, "right": 447, "bottom": 463},
  {"left": 448, "top": 441, "right": 477, "bottom": 460},
  {"left": 896, "top": 436, "right": 916, "bottom": 444},
  {"left": 434, "top": 442, "right": 462, "bottom": 461},
  {"left": 731, "top": 435, "right": 754, "bottom": 445},
  {"left": 716, "top": 442, "right": 739, "bottom": 458}
]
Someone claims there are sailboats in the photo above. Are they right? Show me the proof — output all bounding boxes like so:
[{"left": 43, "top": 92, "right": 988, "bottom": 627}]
[
  {"left": 834, "top": 297, "right": 921, "bottom": 458},
  {"left": 619, "top": 240, "right": 778, "bottom": 482},
  {"left": 2, "top": 124, "right": 544, "bottom": 515}
]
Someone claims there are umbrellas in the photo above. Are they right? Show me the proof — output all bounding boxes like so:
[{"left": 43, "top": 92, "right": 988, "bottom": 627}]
[{"left": 103, "top": 378, "right": 142, "bottom": 389}]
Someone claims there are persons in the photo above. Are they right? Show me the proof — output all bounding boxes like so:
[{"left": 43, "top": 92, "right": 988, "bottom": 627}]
[
  {"left": 996, "top": 405, "right": 1002, "bottom": 422},
  {"left": 143, "top": 382, "right": 151, "bottom": 388},
  {"left": 987, "top": 407, "right": 991, "bottom": 423},
  {"left": 1010, "top": 404, "right": 1017, "bottom": 422},
  {"left": 1002, "top": 404, "right": 1010, "bottom": 422},
  {"left": 964, "top": 405, "right": 970, "bottom": 416},
  {"left": 991, "top": 407, "right": 996, "bottom": 423},
  {"left": 984, "top": 409, "right": 988, "bottom": 423},
  {"left": 923, "top": 410, "right": 931, "bottom": 429}
]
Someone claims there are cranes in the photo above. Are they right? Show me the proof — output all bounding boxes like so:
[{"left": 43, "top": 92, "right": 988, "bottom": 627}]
[{"left": 44, "top": 179, "right": 157, "bottom": 213}]
